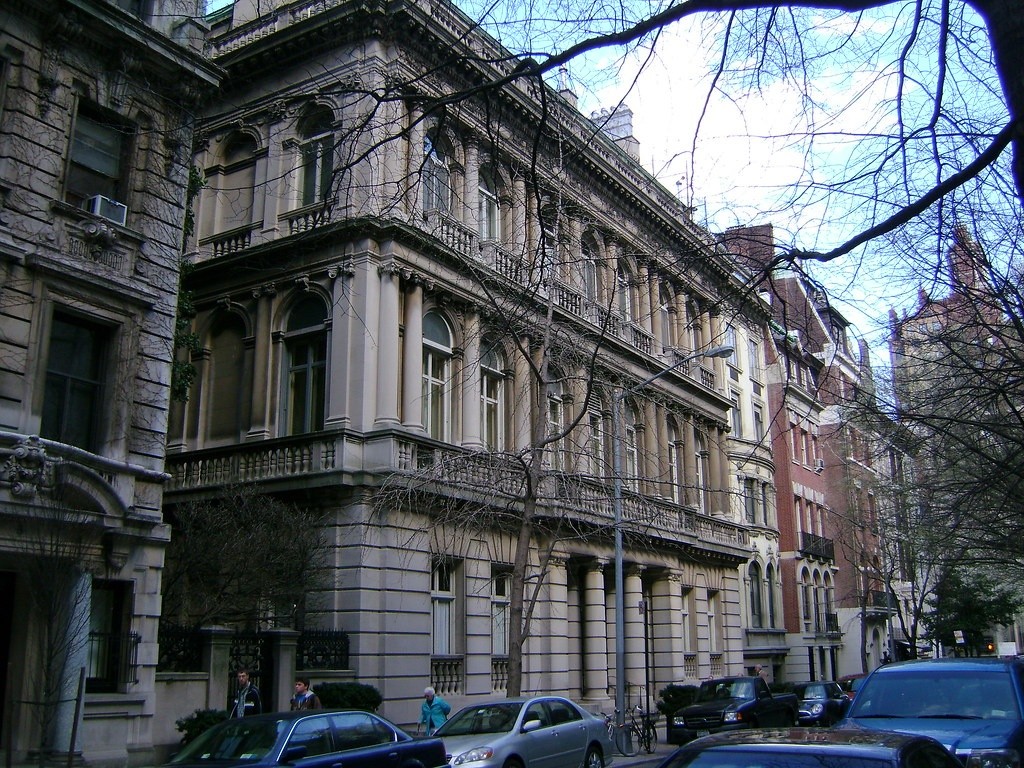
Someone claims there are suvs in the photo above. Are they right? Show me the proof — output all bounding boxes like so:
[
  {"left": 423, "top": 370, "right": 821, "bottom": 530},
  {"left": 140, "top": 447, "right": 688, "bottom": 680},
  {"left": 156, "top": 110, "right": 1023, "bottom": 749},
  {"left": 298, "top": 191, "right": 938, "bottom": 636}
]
[{"left": 833, "top": 657, "right": 1023, "bottom": 768}]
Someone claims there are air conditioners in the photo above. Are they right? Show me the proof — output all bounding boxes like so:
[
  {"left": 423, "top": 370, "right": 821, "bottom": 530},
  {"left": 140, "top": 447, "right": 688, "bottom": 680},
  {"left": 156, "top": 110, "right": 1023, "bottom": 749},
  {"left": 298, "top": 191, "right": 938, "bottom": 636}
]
[{"left": 85, "top": 195, "right": 128, "bottom": 228}]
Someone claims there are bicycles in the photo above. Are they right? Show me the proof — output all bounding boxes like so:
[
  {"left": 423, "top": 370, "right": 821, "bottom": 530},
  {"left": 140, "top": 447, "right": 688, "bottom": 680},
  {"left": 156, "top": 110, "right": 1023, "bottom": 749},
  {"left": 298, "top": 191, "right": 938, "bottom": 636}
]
[{"left": 601, "top": 704, "right": 658, "bottom": 758}]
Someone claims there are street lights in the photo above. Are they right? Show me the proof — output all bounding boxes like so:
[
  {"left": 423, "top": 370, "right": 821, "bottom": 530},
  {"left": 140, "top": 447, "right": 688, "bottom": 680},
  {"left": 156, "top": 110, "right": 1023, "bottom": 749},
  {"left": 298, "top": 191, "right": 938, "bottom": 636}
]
[{"left": 608, "top": 345, "right": 738, "bottom": 751}]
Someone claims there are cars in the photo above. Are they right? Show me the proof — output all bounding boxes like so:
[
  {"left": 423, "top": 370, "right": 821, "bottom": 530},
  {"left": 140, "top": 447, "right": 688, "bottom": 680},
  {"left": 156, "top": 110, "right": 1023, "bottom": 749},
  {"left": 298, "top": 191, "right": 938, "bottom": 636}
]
[
  {"left": 660, "top": 727, "right": 965, "bottom": 768},
  {"left": 159, "top": 707, "right": 453, "bottom": 768},
  {"left": 791, "top": 679, "right": 853, "bottom": 729},
  {"left": 428, "top": 696, "right": 614, "bottom": 768},
  {"left": 837, "top": 673, "right": 877, "bottom": 702}
]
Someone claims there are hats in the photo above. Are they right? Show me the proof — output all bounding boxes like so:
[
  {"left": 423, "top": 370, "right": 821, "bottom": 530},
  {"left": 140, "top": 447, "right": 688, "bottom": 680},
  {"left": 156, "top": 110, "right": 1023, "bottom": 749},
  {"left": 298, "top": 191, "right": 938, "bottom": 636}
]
[{"left": 755, "top": 664, "right": 762, "bottom": 670}]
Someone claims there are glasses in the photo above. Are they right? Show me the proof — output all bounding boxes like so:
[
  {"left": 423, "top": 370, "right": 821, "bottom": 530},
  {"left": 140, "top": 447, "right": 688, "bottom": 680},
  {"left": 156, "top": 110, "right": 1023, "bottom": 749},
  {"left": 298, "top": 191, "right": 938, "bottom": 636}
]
[{"left": 425, "top": 695, "right": 433, "bottom": 697}]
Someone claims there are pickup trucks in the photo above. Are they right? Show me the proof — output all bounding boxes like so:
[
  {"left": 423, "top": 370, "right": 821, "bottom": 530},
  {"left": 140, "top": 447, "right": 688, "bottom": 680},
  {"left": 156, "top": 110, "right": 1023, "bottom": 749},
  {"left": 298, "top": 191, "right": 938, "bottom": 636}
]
[{"left": 664, "top": 674, "right": 800, "bottom": 745}]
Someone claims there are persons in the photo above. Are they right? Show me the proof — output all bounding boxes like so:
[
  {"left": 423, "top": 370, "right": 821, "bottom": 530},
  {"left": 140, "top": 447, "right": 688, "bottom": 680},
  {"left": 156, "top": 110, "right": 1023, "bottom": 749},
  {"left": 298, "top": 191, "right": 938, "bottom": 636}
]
[
  {"left": 755, "top": 664, "right": 769, "bottom": 685},
  {"left": 417, "top": 687, "right": 451, "bottom": 736},
  {"left": 290, "top": 676, "right": 319, "bottom": 710},
  {"left": 718, "top": 688, "right": 731, "bottom": 700},
  {"left": 229, "top": 668, "right": 262, "bottom": 720}
]
